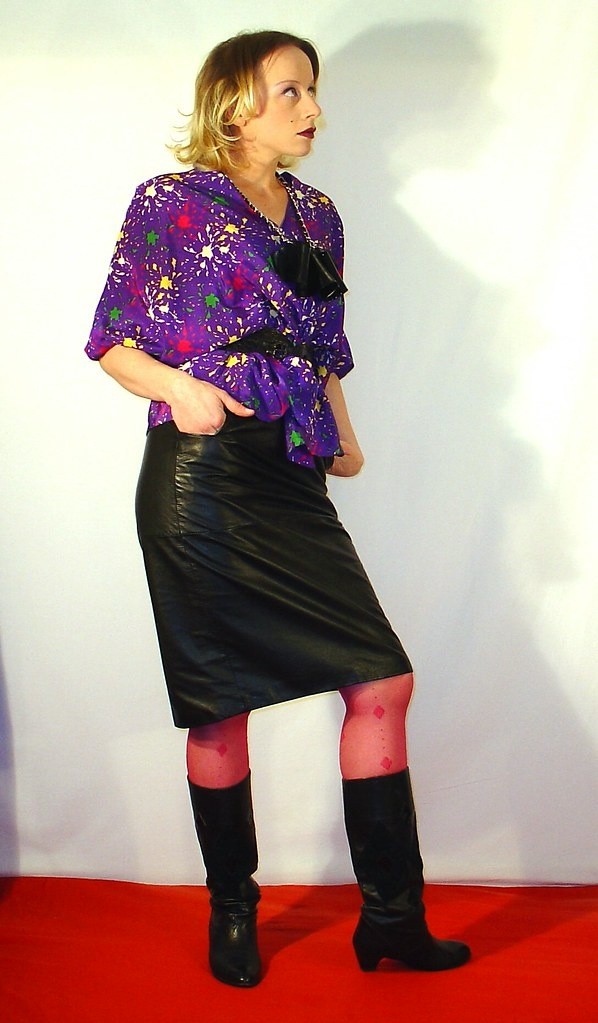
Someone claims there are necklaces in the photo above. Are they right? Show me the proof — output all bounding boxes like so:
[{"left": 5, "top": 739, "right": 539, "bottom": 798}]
[{"left": 234, "top": 172, "right": 315, "bottom": 247}]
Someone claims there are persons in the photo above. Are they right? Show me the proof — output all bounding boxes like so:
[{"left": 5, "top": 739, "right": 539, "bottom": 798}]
[{"left": 85, "top": 30, "right": 474, "bottom": 990}]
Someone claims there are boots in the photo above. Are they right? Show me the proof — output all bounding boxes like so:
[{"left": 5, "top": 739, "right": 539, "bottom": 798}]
[
  {"left": 187, "top": 768, "right": 261, "bottom": 987},
  {"left": 343, "top": 766, "right": 471, "bottom": 972}
]
